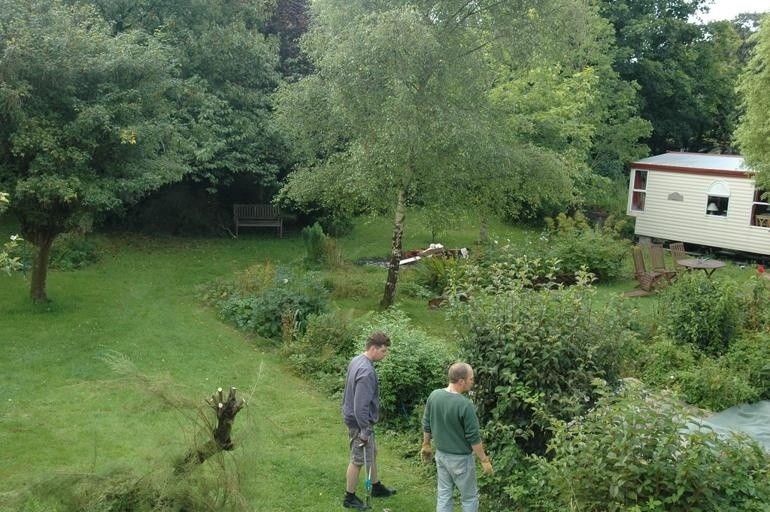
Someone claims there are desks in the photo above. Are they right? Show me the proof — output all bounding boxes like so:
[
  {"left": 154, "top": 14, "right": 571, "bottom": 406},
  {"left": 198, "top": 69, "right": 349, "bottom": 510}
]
[{"left": 677, "top": 258, "right": 726, "bottom": 290}]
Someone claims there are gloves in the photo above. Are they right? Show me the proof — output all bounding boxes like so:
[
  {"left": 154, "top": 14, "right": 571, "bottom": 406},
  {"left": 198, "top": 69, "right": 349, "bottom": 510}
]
[
  {"left": 420, "top": 442, "right": 433, "bottom": 464},
  {"left": 481, "top": 456, "right": 494, "bottom": 477}
]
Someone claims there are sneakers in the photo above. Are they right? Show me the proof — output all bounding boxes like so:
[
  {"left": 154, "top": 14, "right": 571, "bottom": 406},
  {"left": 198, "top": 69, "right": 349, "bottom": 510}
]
[
  {"left": 371, "top": 485, "right": 396, "bottom": 497},
  {"left": 344, "top": 493, "right": 371, "bottom": 509}
]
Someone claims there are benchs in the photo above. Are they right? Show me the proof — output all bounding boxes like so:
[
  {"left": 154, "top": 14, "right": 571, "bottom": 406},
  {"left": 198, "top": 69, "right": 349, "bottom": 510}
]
[{"left": 233, "top": 204, "right": 283, "bottom": 239}]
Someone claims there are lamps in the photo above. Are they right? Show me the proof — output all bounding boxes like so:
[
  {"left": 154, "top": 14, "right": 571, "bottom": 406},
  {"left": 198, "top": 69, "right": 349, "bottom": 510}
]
[{"left": 707, "top": 203, "right": 718, "bottom": 215}]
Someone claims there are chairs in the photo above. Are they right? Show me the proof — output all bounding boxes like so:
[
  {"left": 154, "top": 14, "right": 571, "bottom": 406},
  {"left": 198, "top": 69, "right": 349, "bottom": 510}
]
[
  {"left": 618, "top": 242, "right": 689, "bottom": 297},
  {"left": 755, "top": 215, "right": 770, "bottom": 227}
]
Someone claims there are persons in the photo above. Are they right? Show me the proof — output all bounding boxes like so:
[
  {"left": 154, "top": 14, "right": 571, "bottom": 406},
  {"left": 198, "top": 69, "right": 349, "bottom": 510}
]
[
  {"left": 420, "top": 362, "right": 493, "bottom": 512},
  {"left": 341, "top": 330, "right": 397, "bottom": 509}
]
